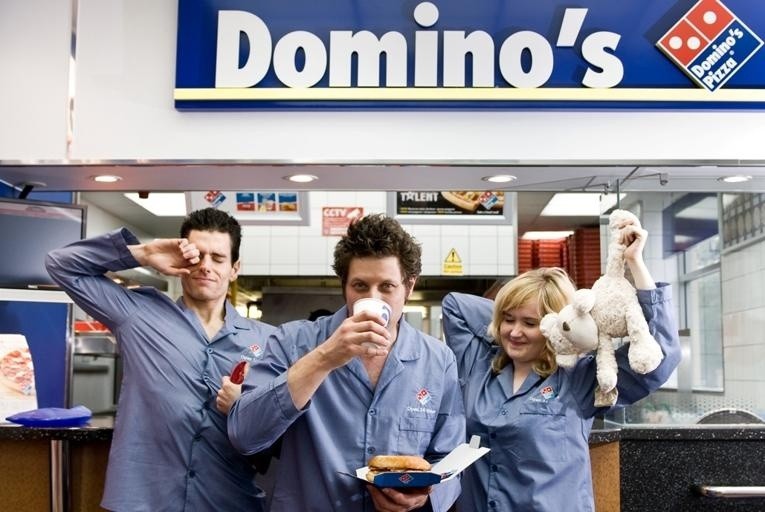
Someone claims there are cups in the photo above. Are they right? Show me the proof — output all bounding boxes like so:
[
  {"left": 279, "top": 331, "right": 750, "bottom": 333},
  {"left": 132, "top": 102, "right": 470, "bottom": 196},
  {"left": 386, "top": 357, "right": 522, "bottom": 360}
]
[{"left": 353, "top": 298, "right": 392, "bottom": 356}]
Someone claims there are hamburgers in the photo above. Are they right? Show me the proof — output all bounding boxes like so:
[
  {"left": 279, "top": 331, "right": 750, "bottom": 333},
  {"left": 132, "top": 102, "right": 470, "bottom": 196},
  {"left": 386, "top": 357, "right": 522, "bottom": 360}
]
[{"left": 366, "top": 455, "right": 432, "bottom": 483}]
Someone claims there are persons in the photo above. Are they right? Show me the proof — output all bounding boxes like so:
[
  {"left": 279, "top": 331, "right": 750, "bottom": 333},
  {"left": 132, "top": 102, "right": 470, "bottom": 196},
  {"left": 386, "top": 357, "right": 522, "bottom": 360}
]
[
  {"left": 441, "top": 220, "right": 684, "bottom": 511},
  {"left": 224, "top": 211, "right": 471, "bottom": 512},
  {"left": 43, "top": 207, "right": 280, "bottom": 511}
]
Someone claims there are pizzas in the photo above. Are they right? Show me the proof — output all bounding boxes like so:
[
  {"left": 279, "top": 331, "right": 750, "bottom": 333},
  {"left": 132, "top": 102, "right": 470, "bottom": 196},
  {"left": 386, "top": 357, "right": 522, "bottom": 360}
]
[{"left": 0, "top": 347, "right": 35, "bottom": 395}]
